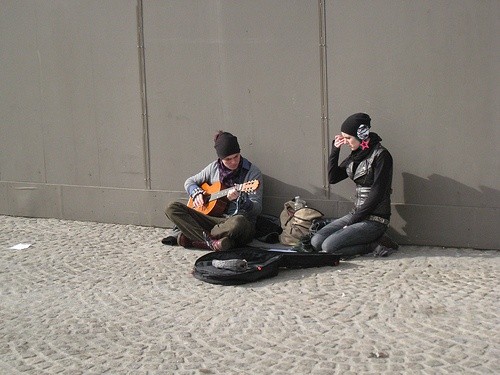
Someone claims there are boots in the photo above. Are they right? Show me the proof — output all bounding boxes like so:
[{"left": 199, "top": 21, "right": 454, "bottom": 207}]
[
  {"left": 193, "top": 231, "right": 231, "bottom": 251},
  {"left": 177, "top": 231, "right": 192, "bottom": 247}
]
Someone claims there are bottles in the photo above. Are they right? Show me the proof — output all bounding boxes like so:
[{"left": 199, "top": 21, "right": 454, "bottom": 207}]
[{"left": 294, "top": 196, "right": 303, "bottom": 211}]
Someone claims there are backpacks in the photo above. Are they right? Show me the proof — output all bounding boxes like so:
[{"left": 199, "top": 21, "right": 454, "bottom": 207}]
[
  {"left": 279, "top": 197, "right": 324, "bottom": 246},
  {"left": 191, "top": 247, "right": 282, "bottom": 285}
]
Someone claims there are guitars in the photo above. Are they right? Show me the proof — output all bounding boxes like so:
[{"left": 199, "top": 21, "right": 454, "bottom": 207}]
[{"left": 186, "top": 178, "right": 261, "bottom": 217}]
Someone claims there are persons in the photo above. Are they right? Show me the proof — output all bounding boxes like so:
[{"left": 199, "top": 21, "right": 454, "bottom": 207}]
[
  {"left": 310, "top": 112, "right": 400, "bottom": 257},
  {"left": 160, "top": 130, "right": 264, "bottom": 253}
]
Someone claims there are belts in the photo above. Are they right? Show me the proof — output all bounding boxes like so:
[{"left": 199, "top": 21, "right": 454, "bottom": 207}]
[{"left": 365, "top": 214, "right": 390, "bottom": 227}]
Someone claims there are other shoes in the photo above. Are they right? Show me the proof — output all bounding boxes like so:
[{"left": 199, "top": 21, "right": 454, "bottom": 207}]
[{"left": 380, "top": 232, "right": 399, "bottom": 250}]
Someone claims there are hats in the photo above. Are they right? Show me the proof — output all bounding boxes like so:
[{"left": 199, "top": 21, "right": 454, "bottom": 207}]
[
  {"left": 214, "top": 130, "right": 241, "bottom": 160},
  {"left": 340, "top": 112, "right": 372, "bottom": 142}
]
